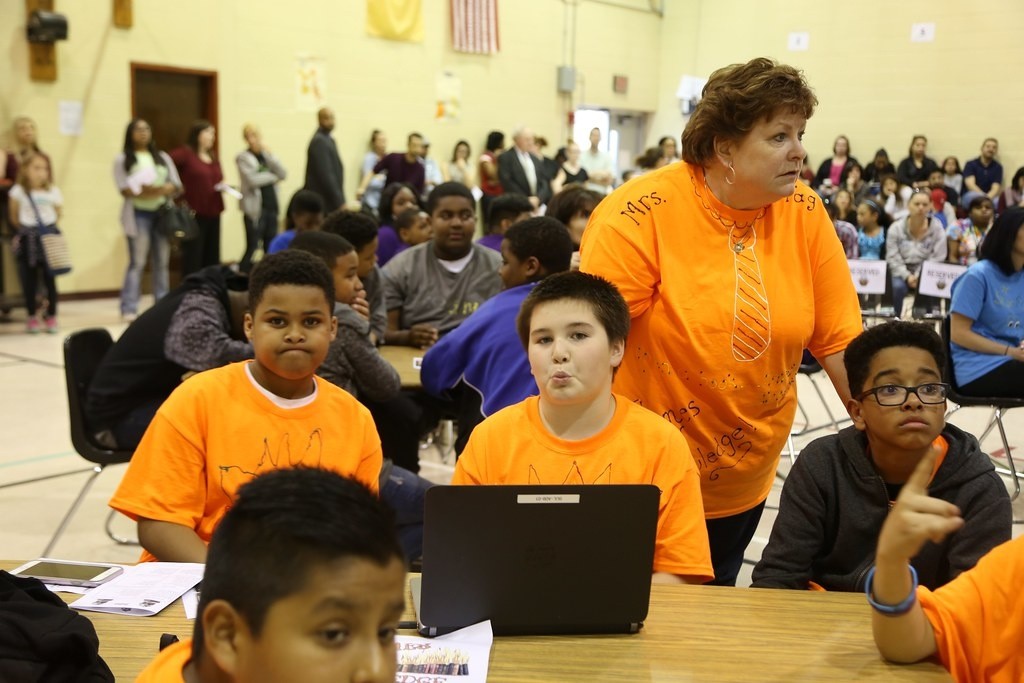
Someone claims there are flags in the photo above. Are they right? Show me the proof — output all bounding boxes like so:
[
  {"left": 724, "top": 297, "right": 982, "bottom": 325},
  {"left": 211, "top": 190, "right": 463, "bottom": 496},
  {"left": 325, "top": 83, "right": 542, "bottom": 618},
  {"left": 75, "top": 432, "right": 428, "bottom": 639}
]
[
  {"left": 366, "top": 0, "right": 425, "bottom": 44},
  {"left": 450, "top": 0, "right": 500, "bottom": 56}
]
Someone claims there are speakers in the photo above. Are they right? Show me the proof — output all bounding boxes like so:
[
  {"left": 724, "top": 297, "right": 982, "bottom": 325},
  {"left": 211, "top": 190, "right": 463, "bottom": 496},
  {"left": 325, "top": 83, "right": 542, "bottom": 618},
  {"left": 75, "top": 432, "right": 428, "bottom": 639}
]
[{"left": 557, "top": 65, "right": 576, "bottom": 93}]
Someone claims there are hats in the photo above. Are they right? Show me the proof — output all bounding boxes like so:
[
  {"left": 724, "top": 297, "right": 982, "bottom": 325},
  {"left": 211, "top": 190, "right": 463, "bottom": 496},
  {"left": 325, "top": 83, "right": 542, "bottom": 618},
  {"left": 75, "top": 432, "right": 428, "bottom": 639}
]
[{"left": 931, "top": 189, "right": 946, "bottom": 214}]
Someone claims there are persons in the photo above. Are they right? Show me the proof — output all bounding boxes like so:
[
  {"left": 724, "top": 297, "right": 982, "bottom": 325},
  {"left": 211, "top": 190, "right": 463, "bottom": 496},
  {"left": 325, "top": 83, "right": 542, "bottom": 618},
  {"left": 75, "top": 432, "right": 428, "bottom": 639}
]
[
  {"left": 749, "top": 322, "right": 1013, "bottom": 592},
  {"left": 577, "top": 58, "right": 866, "bottom": 589},
  {"left": 107, "top": 251, "right": 382, "bottom": 563},
  {"left": 797, "top": 135, "right": 1024, "bottom": 400},
  {"left": 450, "top": 268, "right": 716, "bottom": 587},
  {"left": 134, "top": 465, "right": 407, "bottom": 683},
  {"left": 866, "top": 445, "right": 1024, "bottom": 683},
  {"left": 0, "top": 107, "right": 681, "bottom": 569}
]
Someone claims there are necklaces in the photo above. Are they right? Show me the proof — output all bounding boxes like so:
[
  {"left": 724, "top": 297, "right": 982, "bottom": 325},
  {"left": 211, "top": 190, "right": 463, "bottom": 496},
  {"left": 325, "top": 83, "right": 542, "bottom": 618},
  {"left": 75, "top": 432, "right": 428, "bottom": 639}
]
[{"left": 704, "top": 176, "right": 760, "bottom": 254}]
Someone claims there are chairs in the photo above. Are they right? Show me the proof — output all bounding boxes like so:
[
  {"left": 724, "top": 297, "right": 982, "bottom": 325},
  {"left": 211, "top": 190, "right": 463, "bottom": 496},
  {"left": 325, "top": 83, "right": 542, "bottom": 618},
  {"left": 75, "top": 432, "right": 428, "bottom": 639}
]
[
  {"left": 43, "top": 328, "right": 141, "bottom": 562},
  {"left": 774, "top": 260, "right": 1023, "bottom": 522}
]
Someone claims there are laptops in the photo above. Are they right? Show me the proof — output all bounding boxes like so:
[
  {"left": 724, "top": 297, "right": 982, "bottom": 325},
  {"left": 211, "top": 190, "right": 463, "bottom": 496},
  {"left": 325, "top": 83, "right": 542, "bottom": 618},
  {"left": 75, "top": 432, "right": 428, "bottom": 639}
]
[{"left": 408, "top": 484, "right": 659, "bottom": 635}]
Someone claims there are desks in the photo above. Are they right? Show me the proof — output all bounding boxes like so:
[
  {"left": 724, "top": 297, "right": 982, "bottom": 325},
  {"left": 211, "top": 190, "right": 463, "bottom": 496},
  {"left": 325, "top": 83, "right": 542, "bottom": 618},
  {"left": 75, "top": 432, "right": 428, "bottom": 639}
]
[
  {"left": 0, "top": 559, "right": 957, "bottom": 683},
  {"left": 377, "top": 343, "right": 436, "bottom": 389}
]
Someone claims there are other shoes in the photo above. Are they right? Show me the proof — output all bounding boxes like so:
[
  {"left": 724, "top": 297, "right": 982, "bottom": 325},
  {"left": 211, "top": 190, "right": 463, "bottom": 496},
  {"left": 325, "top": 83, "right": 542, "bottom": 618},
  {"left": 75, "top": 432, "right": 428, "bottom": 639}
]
[
  {"left": 45, "top": 316, "right": 59, "bottom": 332},
  {"left": 28, "top": 318, "right": 38, "bottom": 333},
  {"left": 124, "top": 313, "right": 134, "bottom": 320}
]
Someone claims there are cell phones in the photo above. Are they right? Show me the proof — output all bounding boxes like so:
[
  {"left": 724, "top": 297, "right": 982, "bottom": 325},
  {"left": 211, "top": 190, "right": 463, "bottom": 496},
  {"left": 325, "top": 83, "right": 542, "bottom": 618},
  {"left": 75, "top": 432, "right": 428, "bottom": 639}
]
[{"left": 9, "top": 560, "right": 124, "bottom": 588}]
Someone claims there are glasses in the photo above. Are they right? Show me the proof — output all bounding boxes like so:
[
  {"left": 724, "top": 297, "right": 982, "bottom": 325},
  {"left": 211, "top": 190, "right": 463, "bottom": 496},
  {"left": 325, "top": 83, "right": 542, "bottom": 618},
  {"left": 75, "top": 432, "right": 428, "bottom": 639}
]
[{"left": 855, "top": 382, "right": 951, "bottom": 407}]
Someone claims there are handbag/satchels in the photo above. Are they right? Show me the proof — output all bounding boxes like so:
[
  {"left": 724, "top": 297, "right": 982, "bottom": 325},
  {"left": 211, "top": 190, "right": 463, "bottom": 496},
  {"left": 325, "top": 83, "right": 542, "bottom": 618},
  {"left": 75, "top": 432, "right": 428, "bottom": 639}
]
[
  {"left": 158, "top": 192, "right": 201, "bottom": 245},
  {"left": 38, "top": 232, "right": 73, "bottom": 275}
]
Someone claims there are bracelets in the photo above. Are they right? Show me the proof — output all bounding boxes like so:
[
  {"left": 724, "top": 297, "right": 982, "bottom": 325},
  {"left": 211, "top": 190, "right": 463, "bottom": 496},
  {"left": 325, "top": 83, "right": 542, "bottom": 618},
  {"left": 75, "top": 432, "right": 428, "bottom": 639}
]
[{"left": 865, "top": 563, "right": 918, "bottom": 617}]
